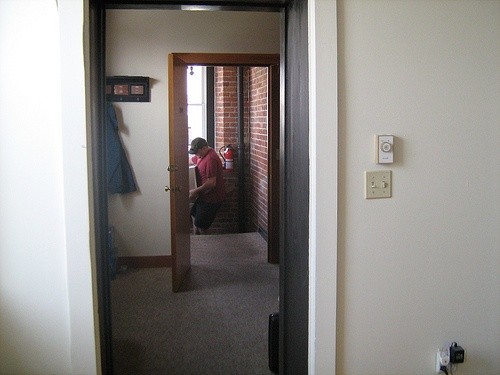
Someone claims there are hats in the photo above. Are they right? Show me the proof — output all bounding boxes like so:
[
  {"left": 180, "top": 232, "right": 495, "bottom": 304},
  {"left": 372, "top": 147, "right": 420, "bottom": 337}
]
[{"left": 189, "top": 137, "right": 207, "bottom": 154}]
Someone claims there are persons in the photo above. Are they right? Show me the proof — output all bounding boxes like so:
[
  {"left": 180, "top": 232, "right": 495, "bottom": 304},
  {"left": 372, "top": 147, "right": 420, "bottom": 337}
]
[{"left": 188, "top": 136, "right": 225, "bottom": 235}]
